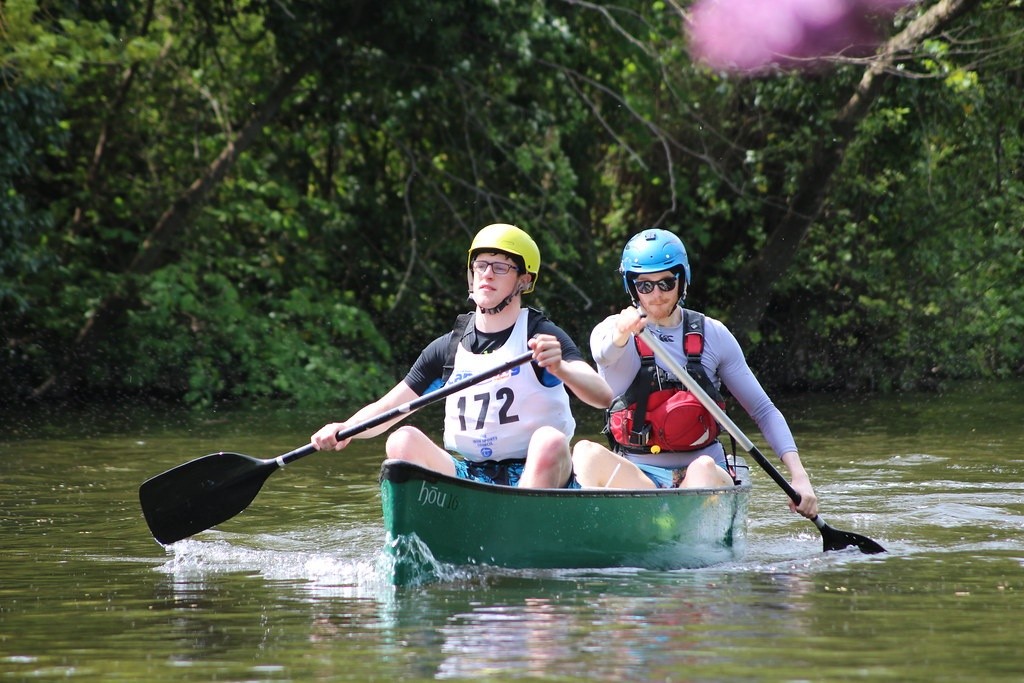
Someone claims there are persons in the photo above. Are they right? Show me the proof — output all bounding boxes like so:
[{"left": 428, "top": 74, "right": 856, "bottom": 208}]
[
  {"left": 309, "top": 224, "right": 613, "bottom": 489},
  {"left": 572, "top": 230, "right": 817, "bottom": 519}
]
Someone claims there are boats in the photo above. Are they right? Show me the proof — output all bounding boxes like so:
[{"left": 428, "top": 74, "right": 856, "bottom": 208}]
[{"left": 378, "top": 454, "right": 754, "bottom": 583}]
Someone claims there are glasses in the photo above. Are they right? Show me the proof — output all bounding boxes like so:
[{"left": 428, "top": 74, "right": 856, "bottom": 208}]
[
  {"left": 633, "top": 273, "right": 679, "bottom": 294},
  {"left": 471, "top": 260, "right": 520, "bottom": 274}
]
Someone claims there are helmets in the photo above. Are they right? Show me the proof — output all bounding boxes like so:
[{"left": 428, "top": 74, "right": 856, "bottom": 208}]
[
  {"left": 468, "top": 224, "right": 540, "bottom": 295},
  {"left": 619, "top": 229, "right": 690, "bottom": 294}
]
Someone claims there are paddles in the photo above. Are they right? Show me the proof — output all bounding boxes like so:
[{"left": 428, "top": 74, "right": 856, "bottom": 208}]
[
  {"left": 632, "top": 320, "right": 890, "bottom": 559},
  {"left": 137, "top": 341, "right": 555, "bottom": 548}
]
